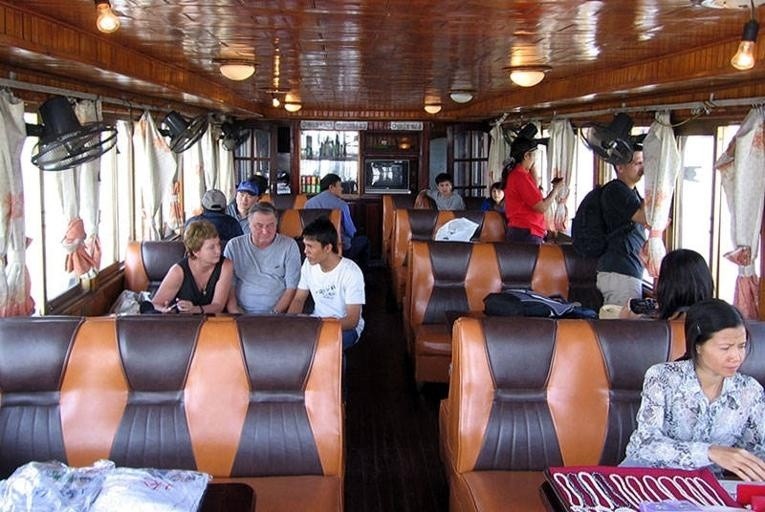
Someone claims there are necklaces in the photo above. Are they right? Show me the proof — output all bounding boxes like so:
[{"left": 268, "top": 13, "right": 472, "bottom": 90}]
[
  {"left": 190, "top": 261, "right": 211, "bottom": 295},
  {"left": 553, "top": 471, "right": 726, "bottom": 512}
]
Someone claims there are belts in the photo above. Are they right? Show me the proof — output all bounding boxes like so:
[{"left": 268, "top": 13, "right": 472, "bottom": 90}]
[{"left": 507, "top": 227, "right": 530, "bottom": 234}]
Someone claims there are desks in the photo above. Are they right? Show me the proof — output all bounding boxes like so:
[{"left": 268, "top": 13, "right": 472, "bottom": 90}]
[
  {"left": 539, "top": 478, "right": 765, "bottom": 512},
  {"left": 1, "top": 483, "right": 257, "bottom": 511}
]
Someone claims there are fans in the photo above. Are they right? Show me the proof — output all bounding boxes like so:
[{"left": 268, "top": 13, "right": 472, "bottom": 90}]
[
  {"left": 216, "top": 121, "right": 250, "bottom": 152},
  {"left": 503, "top": 122, "right": 550, "bottom": 153},
  {"left": 580, "top": 113, "right": 648, "bottom": 165},
  {"left": 158, "top": 110, "right": 209, "bottom": 153},
  {"left": 31, "top": 94, "right": 118, "bottom": 171}
]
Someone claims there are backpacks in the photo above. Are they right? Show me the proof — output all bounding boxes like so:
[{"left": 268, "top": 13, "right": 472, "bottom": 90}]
[
  {"left": 571, "top": 183, "right": 606, "bottom": 261},
  {"left": 482, "top": 287, "right": 598, "bottom": 318}
]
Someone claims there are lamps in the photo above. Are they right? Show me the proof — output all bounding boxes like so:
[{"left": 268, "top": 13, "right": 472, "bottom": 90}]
[
  {"left": 423, "top": 104, "right": 442, "bottom": 114},
  {"left": 284, "top": 102, "right": 303, "bottom": 113},
  {"left": 272, "top": 92, "right": 280, "bottom": 106},
  {"left": 95, "top": 0, "right": 120, "bottom": 33},
  {"left": 449, "top": 91, "right": 476, "bottom": 104},
  {"left": 502, "top": 65, "right": 553, "bottom": 88},
  {"left": 701, "top": 0, "right": 765, "bottom": 71},
  {"left": 209, "top": 59, "right": 259, "bottom": 81}
]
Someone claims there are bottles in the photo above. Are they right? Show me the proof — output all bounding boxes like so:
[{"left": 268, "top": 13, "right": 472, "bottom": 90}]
[
  {"left": 301, "top": 176, "right": 305, "bottom": 193},
  {"left": 306, "top": 176, "right": 310, "bottom": 193},
  {"left": 320, "top": 135, "right": 344, "bottom": 156},
  {"left": 311, "top": 176, "right": 316, "bottom": 193},
  {"left": 316, "top": 176, "right": 321, "bottom": 193}
]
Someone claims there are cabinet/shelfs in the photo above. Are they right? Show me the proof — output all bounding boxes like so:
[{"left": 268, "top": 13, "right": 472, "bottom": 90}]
[{"left": 298, "top": 128, "right": 423, "bottom": 260}]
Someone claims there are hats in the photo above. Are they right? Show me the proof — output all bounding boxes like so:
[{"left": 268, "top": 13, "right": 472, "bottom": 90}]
[
  {"left": 201, "top": 188, "right": 227, "bottom": 211},
  {"left": 237, "top": 180, "right": 259, "bottom": 196}
]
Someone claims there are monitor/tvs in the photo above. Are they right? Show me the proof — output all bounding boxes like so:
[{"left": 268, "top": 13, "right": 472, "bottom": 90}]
[{"left": 360, "top": 156, "right": 413, "bottom": 196}]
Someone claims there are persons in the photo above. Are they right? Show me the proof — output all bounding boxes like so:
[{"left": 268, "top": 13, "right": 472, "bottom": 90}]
[
  {"left": 618, "top": 299, "right": 765, "bottom": 481},
  {"left": 499, "top": 136, "right": 565, "bottom": 245},
  {"left": 186, "top": 173, "right": 270, "bottom": 240},
  {"left": 414, "top": 173, "right": 467, "bottom": 210},
  {"left": 151, "top": 219, "right": 233, "bottom": 315},
  {"left": 304, "top": 174, "right": 372, "bottom": 262},
  {"left": 481, "top": 182, "right": 505, "bottom": 212},
  {"left": 595, "top": 144, "right": 651, "bottom": 307},
  {"left": 223, "top": 201, "right": 302, "bottom": 313},
  {"left": 620, "top": 248, "right": 714, "bottom": 320},
  {"left": 287, "top": 219, "right": 366, "bottom": 373}
]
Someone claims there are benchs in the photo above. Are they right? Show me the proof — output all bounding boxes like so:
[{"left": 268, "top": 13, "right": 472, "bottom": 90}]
[
  {"left": 450, "top": 316, "right": 765, "bottom": 512},
  {"left": 1, "top": 316, "right": 346, "bottom": 512},
  {"left": 438, "top": 363, "right": 452, "bottom": 484},
  {"left": 123, "top": 238, "right": 315, "bottom": 315},
  {"left": 258, "top": 193, "right": 319, "bottom": 210},
  {"left": 391, "top": 208, "right": 509, "bottom": 310},
  {"left": 407, "top": 239, "right": 604, "bottom": 383},
  {"left": 402, "top": 278, "right": 408, "bottom": 345},
  {"left": 193, "top": 208, "right": 345, "bottom": 256},
  {"left": 382, "top": 194, "right": 487, "bottom": 269}
]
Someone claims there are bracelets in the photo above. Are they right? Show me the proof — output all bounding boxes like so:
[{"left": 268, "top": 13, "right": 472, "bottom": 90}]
[
  {"left": 199, "top": 304, "right": 204, "bottom": 314},
  {"left": 271, "top": 308, "right": 280, "bottom": 314}
]
